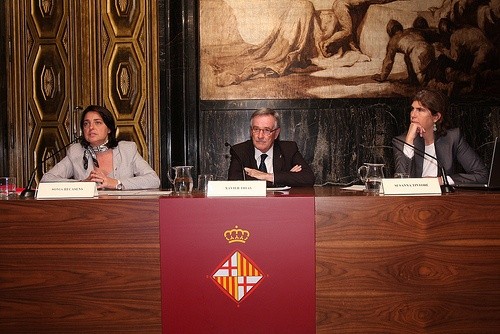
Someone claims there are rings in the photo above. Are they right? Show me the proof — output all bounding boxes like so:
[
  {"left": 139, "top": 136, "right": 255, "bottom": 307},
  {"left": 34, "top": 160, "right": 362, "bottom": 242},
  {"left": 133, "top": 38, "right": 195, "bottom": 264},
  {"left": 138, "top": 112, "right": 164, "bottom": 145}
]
[{"left": 101, "top": 180, "right": 104, "bottom": 184}]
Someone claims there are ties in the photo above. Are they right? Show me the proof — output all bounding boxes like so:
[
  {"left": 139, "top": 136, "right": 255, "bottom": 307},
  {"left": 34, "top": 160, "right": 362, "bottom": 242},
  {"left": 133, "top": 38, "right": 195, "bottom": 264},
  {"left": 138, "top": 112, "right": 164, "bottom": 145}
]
[{"left": 259, "top": 154, "right": 268, "bottom": 173}]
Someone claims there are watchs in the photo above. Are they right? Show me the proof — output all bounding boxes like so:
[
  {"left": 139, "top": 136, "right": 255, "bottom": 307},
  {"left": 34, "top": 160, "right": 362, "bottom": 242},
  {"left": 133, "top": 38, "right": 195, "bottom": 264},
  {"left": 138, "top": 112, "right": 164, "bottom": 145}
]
[{"left": 116, "top": 180, "right": 123, "bottom": 191}]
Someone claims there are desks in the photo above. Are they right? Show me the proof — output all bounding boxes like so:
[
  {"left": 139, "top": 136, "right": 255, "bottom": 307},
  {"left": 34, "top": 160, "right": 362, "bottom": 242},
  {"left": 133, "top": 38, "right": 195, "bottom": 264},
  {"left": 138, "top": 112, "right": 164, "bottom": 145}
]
[{"left": 0, "top": 187, "right": 500, "bottom": 334}]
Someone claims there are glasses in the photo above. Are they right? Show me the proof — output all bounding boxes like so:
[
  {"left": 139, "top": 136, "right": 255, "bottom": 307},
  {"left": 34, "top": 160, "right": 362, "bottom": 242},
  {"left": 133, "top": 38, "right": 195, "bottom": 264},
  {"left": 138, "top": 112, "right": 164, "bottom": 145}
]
[{"left": 250, "top": 127, "right": 279, "bottom": 134}]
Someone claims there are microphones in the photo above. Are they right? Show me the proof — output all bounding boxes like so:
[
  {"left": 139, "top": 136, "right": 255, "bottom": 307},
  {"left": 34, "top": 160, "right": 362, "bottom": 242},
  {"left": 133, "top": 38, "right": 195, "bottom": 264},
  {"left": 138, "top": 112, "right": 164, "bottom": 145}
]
[
  {"left": 20, "top": 135, "right": 84, "bottom": 198},
  {"left": 224, "top": 142, "right": 246, "bottom": 180},
  {"left": 390, "top": 134, "right": 453, "bottom": 192}
]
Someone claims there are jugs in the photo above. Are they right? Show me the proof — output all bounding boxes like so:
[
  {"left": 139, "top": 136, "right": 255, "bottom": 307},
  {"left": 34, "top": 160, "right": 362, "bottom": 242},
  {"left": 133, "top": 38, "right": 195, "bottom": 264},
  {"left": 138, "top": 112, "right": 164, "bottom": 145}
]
[
  {"left": 167, "top": 166, "right": 194, "bottom": 195},
  {"left": 357, "top": 163, "right": 386, "bottom": 193}
]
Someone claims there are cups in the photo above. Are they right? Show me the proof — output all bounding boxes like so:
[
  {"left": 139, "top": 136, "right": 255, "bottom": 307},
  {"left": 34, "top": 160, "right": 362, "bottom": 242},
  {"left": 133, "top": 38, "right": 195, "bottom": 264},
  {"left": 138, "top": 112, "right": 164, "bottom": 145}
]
[
  {"left": 198, "top": 174, "right": 212, "bottom": 193},
  {"left": 394, "top": 173, "right": 409, "bottom": 178},
  {"left": 0, "top": 177, "right": 16, "bottom": 199}
]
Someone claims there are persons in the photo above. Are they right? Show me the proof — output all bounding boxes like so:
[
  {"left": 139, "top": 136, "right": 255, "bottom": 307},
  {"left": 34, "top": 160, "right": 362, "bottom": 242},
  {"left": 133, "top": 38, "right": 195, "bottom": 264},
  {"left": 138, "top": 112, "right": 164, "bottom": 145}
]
[
  {"left": 40, "top": 105, "right": 160, "bottom": 190},
  {"left": 392, "top": 89, "right": 488, "bottom": 186},
  {"left": 228, "top": 108, "right": 315, "bottom": 188}
]
